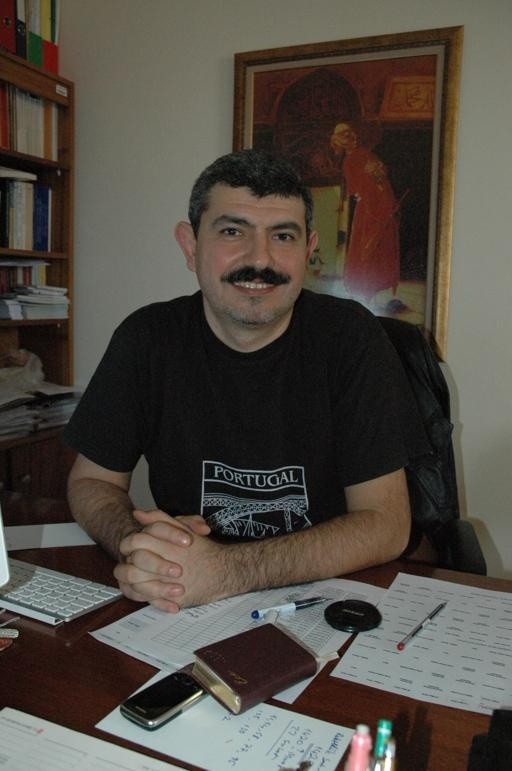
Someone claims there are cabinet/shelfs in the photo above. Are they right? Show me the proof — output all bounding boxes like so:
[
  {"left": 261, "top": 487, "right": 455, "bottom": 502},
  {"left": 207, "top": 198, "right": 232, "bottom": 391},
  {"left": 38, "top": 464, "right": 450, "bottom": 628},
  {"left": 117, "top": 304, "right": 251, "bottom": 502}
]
[{"left": 1, "top": 52, "right": 78, "bottom": 499}]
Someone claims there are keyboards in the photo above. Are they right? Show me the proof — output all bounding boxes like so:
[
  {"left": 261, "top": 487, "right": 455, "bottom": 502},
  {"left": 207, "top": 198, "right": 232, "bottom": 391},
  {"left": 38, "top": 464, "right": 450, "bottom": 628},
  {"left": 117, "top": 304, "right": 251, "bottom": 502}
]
[{"left": 1, "top": 558, "right": 123, "bottom": 628}]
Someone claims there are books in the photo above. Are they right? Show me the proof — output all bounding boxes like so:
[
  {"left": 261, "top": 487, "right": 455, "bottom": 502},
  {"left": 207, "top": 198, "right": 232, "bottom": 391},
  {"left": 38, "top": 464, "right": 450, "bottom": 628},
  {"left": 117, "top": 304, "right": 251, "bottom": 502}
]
[
  {"left": 0, "top": 80, "right": 69, "bottom": 321},
  {"left": 190, "top": 623, "right": 317, "bottom": 716}
]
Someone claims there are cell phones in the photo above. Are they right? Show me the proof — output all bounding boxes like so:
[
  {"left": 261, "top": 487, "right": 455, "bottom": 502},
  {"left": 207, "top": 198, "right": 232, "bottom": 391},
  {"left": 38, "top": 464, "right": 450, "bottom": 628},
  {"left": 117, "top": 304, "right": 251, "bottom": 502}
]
[{"left": 120, "top": 672, "right": 203, "bottom": 729}]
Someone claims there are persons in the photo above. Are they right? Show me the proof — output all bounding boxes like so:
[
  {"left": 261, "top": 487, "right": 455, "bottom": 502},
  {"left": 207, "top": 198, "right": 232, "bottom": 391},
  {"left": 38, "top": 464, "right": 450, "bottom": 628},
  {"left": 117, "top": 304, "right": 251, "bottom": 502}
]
[
  {"left": 328, "top": 123, "right": 406, "bottom": 316},
  {"left": 62, "top": 148, "right": 432, "bottom": 613}
]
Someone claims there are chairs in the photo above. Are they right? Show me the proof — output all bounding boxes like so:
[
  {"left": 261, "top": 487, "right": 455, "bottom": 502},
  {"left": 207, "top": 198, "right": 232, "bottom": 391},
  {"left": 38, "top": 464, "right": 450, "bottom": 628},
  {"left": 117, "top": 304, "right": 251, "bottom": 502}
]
[{"left": 370, "top": 312, "right": 490, "bottom": 576}]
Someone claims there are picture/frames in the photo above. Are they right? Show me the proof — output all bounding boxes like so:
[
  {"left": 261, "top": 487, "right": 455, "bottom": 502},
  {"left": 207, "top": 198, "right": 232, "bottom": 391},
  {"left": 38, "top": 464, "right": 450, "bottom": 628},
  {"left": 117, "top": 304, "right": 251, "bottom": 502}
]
[{"left": 228, "top": 23, "right": 466, "bottom": 362}]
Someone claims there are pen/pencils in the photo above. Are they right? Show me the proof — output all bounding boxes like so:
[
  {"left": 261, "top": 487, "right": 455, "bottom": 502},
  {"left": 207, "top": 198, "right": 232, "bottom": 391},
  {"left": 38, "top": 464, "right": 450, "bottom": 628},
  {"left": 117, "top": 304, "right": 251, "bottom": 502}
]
[
  {"left": 350, "top": 723, "right": 395, "bottom": 770},
  {"left": 252, "top": 597, "right": 327, "bottom": 619},
  {"left": 398, "top": 601, "right": 448, "bottom": 650}
]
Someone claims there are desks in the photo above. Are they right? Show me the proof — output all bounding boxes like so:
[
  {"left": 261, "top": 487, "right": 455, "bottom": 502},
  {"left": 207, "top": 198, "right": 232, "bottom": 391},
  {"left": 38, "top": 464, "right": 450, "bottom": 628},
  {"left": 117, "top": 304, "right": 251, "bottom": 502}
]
[{"left": 0, "top": 499, "right": 511, "bottom": 771}]
